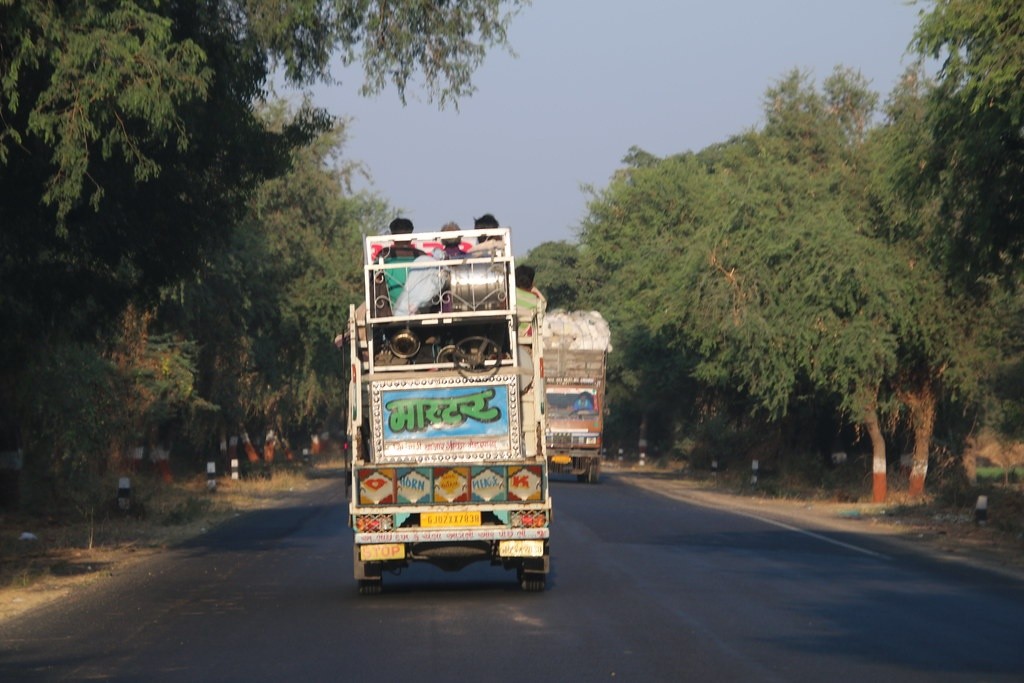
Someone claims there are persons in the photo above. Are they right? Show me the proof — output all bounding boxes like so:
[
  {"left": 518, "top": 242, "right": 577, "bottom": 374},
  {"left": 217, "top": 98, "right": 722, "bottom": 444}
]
[
  {"left": 573, "top": 392, "right": 595, "bottom": 411},
  {"left": 371, "top": 217, "right": 434, "bottom": 312},
  {"left": 468, "top": 211, "right": 510, "bottom": 359},
  {"left": 434, "top": 223, "right": 468, "bottom": 339},
  {"left": 515, "top": 262, "right": 548, "bottom": 393}
]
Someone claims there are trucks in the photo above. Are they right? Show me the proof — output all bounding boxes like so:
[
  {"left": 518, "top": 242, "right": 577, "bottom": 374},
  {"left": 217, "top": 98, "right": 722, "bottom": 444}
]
[
  {"left": 545, "top": 349, "right": 610, "bottom": 484},
  {"left": 347, "top": 303, "right": 552, "bottom": 598}
]
